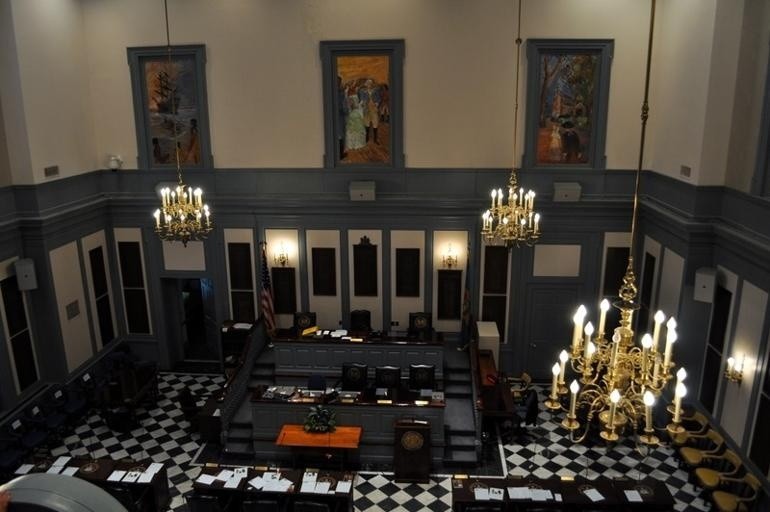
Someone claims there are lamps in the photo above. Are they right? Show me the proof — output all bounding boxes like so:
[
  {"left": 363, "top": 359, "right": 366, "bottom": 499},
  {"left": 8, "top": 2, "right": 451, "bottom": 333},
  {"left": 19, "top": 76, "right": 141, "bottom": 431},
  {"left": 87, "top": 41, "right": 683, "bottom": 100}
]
[
  {"left": 152, "top": 0, "right": 213, "bottom": 245},
  {"left": 549, "top": 1, "right": 688, "bottom": 447},
  {"left": 481, "top": 1, "right": 541, "bottom": 249}
]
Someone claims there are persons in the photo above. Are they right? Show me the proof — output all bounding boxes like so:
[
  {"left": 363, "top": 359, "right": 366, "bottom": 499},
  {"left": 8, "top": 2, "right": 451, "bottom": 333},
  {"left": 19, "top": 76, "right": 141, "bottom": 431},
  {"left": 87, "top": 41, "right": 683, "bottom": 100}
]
[
  {"left": 336, "top": 76, "right": 390, "bottom": 164},
  {"left": 152, "top": 116, "right": 202, "bottom": 167},
  {"left": 546, "top": 89, "right": 591, "bottom": 162}
]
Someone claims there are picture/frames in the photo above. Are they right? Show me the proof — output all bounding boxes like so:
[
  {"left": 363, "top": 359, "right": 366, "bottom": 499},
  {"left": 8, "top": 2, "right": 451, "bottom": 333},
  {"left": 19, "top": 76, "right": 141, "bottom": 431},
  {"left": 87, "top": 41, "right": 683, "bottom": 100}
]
[
  {"left": 521, "top": 39, "right": 614, "bottom": 173},
  {"left": 127, "top": 44, "right": 214, "bottom": 173},
  {"left": 320, "top": 40, "right": 405, "bottom": 169}
]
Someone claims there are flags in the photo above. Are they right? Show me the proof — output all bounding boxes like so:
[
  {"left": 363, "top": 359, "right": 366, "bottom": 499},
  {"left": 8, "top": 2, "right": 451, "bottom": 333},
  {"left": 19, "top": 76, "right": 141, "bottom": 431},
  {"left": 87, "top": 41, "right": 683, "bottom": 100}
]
[
  {"left": 457, "top": 229, "right": 477, "bottom": 351},
  {"left": 257, "top": 240, "right": 277, "bottom": 338}
]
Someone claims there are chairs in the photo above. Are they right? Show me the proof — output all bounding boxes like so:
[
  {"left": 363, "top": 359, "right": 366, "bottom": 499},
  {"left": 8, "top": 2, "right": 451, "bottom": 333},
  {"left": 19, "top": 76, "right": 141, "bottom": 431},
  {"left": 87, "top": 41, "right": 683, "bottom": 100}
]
[
  {"left": 666, "top": 411, "right": 763, "bottom": 512},
  {"left": 177, "top": 386, "right": 203, "bottom": 437},
  {"left": 508, "top": 373, "right": 537, "bottom": 436},
  {"left": 293, "top": 311, "right": 432, "bottom": 340},
  {"left": 308, "top": 363, "right": 438, "bottom": 394},
  {"left": 1, "top": 384, "right": 88, "bottom": 467}
]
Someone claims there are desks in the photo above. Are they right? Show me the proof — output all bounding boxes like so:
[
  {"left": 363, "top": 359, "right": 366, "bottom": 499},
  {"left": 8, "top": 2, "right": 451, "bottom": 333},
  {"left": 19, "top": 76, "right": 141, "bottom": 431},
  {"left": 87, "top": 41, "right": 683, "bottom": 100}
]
[
  {"left": 274, "top": 423, "right": 363, "bottom": 457},
  {"left": 198, "top": 352, "right": 256, "bottom": 447},
  {"left": 10, "top": 456, "right": 171, "bottom": 512},
  {"left": 192, "top": 466, "right": 355, "bottom": 512},
  {"left": 476, "top": 350, "right": 516, "bottom": 419},
  {"left": 451, "top": 478, "right": 676, "bottom": 512}
]
